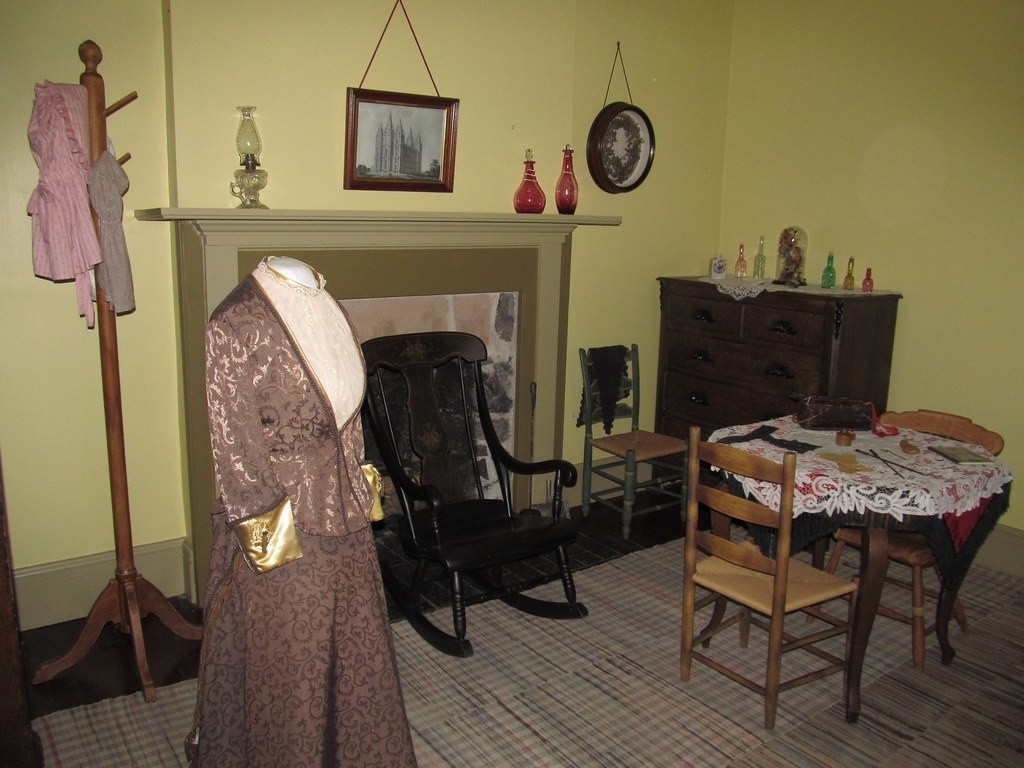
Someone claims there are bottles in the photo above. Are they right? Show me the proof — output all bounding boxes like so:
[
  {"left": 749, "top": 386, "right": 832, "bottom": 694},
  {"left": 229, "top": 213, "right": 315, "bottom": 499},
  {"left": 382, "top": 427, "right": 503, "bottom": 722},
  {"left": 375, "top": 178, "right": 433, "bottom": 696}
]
[
  {"left": 863, "top": 269, "right": 874, "bottom": 294},
  {"left": 710, "top": 253, "right": 728, "bottom": 280},
  {"left": 555, "top": 144, "right": 578, "bottom": 215},
  {"left": 843, "top": 257, "right": 855, "bottom": 291},
  {"left": 735, "top": 244, "right": 747, "bottom": 278},
  {"left": 514, "top": 148, "right": 546, "bottom": 214},
  {"left": 820, "top": 252, "right": 836, "bottom": 289},
  {"left": 754, "top": 236, "right": 767, "bottom": 279}
]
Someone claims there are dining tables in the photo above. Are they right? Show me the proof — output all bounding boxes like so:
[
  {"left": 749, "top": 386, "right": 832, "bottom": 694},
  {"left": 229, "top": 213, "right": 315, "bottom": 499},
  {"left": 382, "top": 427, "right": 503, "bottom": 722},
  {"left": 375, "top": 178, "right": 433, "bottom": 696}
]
[{"left": 701, "top": 412, "right": 1014, "bottom": 726}]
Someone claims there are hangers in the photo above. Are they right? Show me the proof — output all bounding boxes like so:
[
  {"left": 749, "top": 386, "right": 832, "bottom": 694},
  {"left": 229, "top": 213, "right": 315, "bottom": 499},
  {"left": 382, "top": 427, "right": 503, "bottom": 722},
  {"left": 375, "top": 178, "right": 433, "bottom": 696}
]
[{"left": 13, "top": 40, "right": 205, "bottom": 705}]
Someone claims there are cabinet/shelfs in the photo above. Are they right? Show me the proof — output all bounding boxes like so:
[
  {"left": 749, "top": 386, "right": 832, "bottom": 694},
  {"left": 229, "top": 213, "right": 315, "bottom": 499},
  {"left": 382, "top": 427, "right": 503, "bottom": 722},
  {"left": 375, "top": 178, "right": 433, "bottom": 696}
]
[{"left": 649, "top": 272, "right": 903, "bottom": 518}]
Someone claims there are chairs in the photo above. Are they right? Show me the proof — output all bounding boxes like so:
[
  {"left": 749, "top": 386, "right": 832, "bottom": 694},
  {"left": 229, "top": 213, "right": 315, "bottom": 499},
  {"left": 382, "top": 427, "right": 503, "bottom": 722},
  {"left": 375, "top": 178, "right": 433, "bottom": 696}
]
[
  {"left": 805, "top": 406, "right": 1004, "bottom": 668},
  {"left": 576, "top": 343, "right": 692, "bottom": 540},
  {"left": 683, "top": 425, "right": 860, "bottom": 737},
  {"left": 356, "top": 330, "right": 590, "bottom": 657}
]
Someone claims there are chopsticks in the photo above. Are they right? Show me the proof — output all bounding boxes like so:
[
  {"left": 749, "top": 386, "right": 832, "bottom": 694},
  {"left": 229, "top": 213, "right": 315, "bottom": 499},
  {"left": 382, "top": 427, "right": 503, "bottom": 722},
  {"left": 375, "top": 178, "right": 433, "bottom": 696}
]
[{"left": 855, "top": 448, "right": 926, "bottom": 480}]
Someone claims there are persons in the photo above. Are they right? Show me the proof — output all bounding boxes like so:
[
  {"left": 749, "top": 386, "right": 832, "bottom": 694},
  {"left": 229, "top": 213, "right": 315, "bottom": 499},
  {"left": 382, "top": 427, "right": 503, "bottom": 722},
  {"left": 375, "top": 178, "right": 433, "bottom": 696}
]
[{"left": 182, "top": 255, "right": 420, "bottom": 767}]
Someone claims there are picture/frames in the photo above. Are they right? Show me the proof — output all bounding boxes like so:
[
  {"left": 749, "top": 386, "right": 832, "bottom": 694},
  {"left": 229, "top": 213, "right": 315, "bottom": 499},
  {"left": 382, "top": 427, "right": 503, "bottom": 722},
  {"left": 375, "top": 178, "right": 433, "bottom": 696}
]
[{"left": 343, "top": 86, "right": 459, "bottom": 192}]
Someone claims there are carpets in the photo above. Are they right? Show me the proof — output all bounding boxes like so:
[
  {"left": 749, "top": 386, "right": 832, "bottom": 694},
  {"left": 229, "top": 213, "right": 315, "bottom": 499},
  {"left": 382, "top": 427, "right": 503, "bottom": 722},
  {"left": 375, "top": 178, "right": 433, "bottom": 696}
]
[{"left": 28, "top": 513, "right": 1021, "bottom": 767}]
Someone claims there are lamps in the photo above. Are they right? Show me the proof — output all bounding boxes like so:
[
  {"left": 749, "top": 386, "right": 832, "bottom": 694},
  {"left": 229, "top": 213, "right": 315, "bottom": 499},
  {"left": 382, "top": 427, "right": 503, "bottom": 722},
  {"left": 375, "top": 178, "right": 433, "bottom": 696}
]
[{"left": 228, "top": 105, "right": 272, "bottom": 211}]
